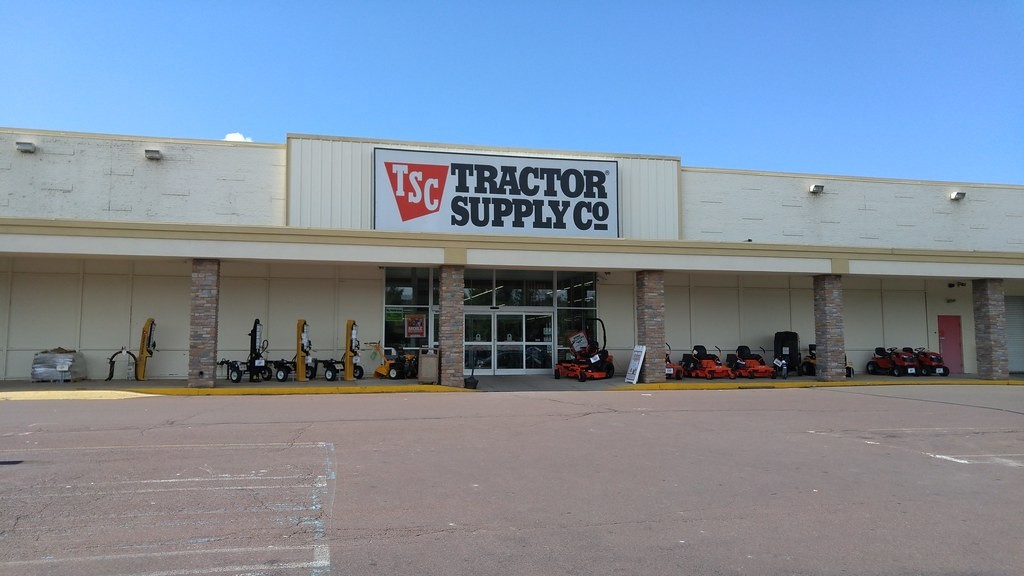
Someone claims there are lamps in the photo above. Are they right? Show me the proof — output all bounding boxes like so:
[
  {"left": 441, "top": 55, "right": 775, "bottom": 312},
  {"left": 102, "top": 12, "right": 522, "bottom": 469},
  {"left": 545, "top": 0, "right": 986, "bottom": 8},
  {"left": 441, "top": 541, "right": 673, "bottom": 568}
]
[
  {"left": 145, "top": 150, "right": 162, "bottom": 160},
  {"left": 15, "top": 142, "right": 37, "bottom": 154},
  {"left": 950, "top": 192, "right": 966, "bottom": 200},
  {"left": 809, "top": 184, "right": 824, "bottom": 193}
]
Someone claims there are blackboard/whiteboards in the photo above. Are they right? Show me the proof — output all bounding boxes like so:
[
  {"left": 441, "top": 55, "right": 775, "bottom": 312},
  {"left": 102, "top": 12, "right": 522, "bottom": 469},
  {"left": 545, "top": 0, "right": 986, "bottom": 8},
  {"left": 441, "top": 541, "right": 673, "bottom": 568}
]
[{"left": 625, "top": 344, "right": 646, "bottom": 384}]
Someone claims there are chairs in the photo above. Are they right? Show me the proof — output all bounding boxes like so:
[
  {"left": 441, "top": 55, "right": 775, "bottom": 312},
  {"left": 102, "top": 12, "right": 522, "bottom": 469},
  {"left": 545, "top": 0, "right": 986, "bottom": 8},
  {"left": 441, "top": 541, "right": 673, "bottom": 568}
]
[
  {"left": 580, "top": 341, "right": 599, "bottom": 358},
  {"left": 694, "top": 346, "right": 712, "bottom": 359},
  {"left": 875, "top": 348, "right": 889, "bottom": 356},
  {"left": 737, "top": 346, "right": 755, "bottom": 360},
  {"left": 902, "top": 347, "right": 914, "bottom": 354}
]
